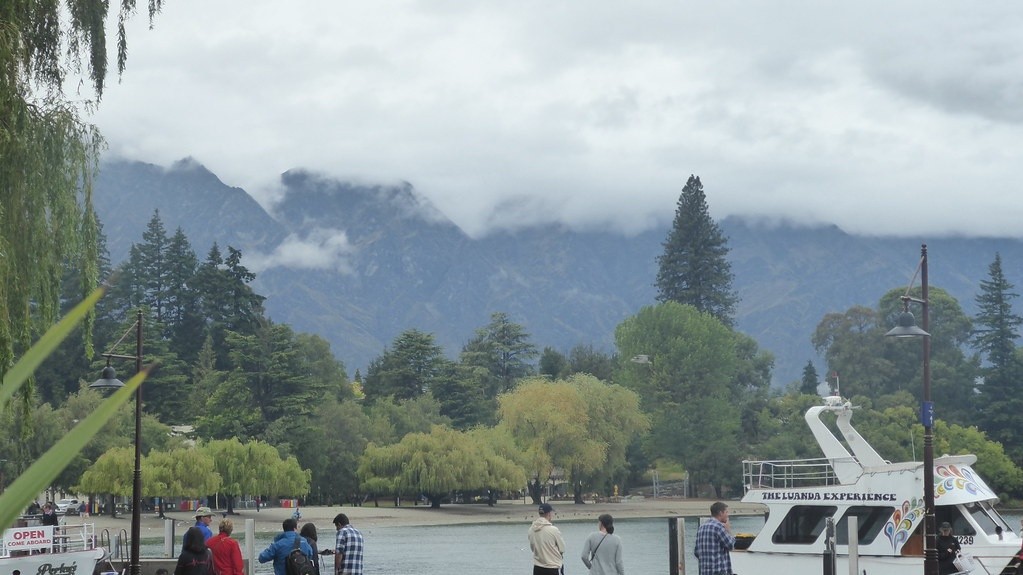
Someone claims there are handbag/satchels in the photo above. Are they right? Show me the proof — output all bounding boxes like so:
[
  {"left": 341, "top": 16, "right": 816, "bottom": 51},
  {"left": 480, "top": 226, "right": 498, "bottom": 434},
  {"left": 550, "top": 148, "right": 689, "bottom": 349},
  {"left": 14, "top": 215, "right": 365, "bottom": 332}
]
[{"left": 953, "top": 550, "right": 975, "bottom": 574}]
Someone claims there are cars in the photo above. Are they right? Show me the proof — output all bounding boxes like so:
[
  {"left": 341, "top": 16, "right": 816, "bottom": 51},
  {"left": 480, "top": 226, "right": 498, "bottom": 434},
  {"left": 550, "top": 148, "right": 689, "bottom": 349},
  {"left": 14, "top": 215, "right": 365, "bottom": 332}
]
[{"left": 53, "top": 499, "right": 80, "bottom": 515}]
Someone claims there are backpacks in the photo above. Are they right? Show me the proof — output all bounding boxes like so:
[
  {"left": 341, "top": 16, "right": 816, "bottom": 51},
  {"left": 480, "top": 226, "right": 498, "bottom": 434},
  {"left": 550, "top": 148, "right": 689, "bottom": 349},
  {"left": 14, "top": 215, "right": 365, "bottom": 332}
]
[{"left": 284, "top": 533, "right": 317, "bottom": 575}]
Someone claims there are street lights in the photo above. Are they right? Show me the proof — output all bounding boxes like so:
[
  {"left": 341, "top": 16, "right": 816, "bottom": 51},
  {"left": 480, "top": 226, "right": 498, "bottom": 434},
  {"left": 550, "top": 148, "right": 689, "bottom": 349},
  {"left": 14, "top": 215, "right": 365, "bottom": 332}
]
[
  {"left": 876, "top": 239, "right": 943, "bottom": 575},
  {"left": 90, "top": 310, "right": 147, "bottom": 575}
]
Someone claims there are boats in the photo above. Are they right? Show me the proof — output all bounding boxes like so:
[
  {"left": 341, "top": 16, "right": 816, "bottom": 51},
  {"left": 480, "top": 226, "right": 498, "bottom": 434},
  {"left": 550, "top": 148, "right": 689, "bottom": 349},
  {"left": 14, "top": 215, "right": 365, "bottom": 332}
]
[{"left": 683, "top": 371, "right": 1023, "bottom": 574}]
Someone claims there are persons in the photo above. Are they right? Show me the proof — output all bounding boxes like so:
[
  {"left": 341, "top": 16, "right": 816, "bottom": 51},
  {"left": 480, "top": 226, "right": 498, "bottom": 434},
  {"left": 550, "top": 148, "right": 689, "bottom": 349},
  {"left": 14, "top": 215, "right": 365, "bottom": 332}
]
[
  {"left": 291, "top": 507, "right": 301, "bottom": 520},
  {"left": 27, "top": 502, "right": 40, "bottom": 514},
  {"left": 580, "top": 514, "right": 625, "bottom": 575},
  {"left": 256, "top": 496, "right": 261, "bottom": 512},
  {"left": 174, "top": 526, "right": 216, "bottom": 575},
  {"left": 300, "top": 522, "right": 320, "bottom": 575},
  {"left": 39, "top": 502, "right": 58, "bottom": 554},
  {"left": 77, "top": 501, "right": 103, "bottom": 517},
  {"left": 332, "top": 513, "right": 364, "bottom": 575},
  {"left": 936, "top": 522, "right": 976, "bottom": 575},
  {"left": 204, "top": 519, "right": 245, "bottom": 575},
  {"left": 693, "top": 502, "right": 736, "bottom": 575},
  {"left": 183, "top": 507, "right": 217, "bottom": 542},
  {"left": 527, "top": 502, "right": 567, "bottom": 575},
  {"left": 258, "top": 519, "right": 314, "bottom": 575}
]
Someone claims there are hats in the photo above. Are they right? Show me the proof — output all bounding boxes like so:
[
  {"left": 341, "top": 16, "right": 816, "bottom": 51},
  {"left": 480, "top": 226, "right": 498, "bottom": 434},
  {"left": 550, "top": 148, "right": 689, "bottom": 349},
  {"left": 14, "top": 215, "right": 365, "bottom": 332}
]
[
  {"left": 941, "top": 522, "right": 951, "bottom": 530},
  {"left": 539, "top": 503, "right": 556, "bottom": 514},
  {"left": 193, "top": 507, "right": 216, "bottom": 518}
]
